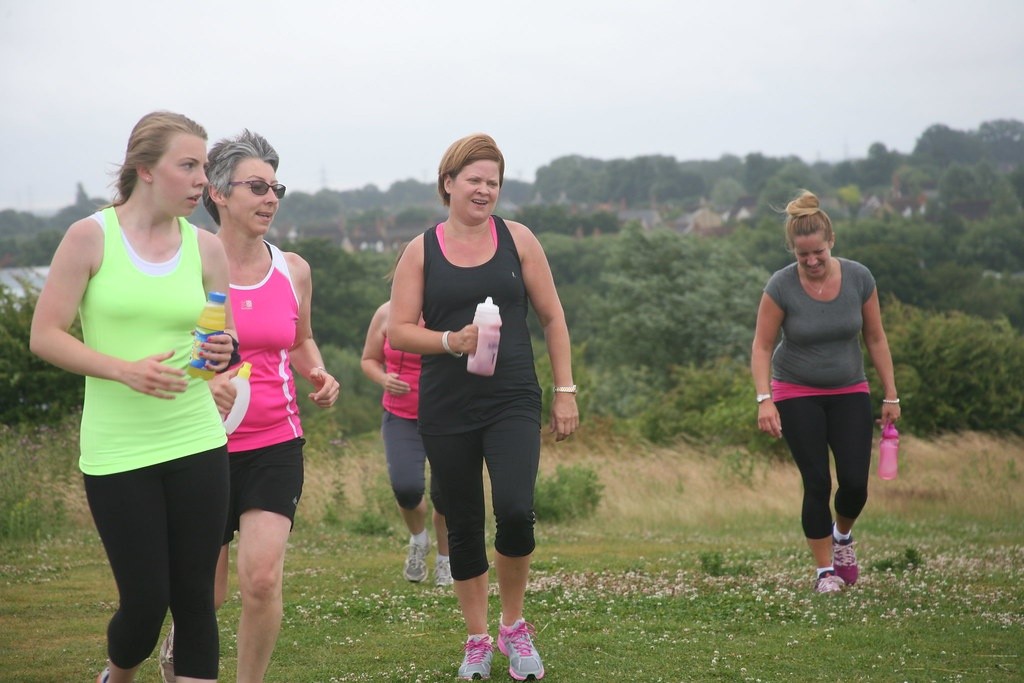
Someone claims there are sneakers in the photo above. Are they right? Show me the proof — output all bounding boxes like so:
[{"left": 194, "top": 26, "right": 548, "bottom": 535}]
[
  {"left": 400, "top": 534, "right": 431, "bottom": 583},
  {"left": 832, "top": 524, "right": 859, "bottom": 585},
  {"left": 434, "top": 558, "right": 454, "bottom": 587},
  {"left": 457, "top": 633, "right": 495, "bottom": 681},
  {"left": 159, "top": 636, "right": 175, "bottom": 683},
  {"left": 495, "top": 611, "right": 544, "bottom": 681},
  {"left": 814, "top": 571, "right": 841, "bottom": 597}
]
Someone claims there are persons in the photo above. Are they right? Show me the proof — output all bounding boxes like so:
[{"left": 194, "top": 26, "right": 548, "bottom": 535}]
[
  {"left": 361, "top": 242, "right": 454, "bottom": 588},
  {"left": 388, "top": 134, "right": 579, "bottom": 681},
  {"left": 29, "top": 111, "right": 240, "bottom": 683},
  {"left": 160, "top": 126, "right": 341, "bottom": 683},
  {"left": 751, "top": 192, "right": 901, "bottom": 597}
]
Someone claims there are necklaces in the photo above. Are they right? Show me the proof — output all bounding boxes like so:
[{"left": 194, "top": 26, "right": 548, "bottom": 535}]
[{"left": 805, "top": 270, "right": 831, "bottom": 296}]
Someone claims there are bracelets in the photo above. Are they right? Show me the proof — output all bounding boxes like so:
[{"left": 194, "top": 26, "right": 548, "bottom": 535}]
[
  {"left": 318, "top": 367, "right": 327, "bottom": 373},
  {"left": 442, "top": 329, "right": 462, "bottom": 358},
  {"left": 883, "top": 399, "right": 900, "bottom": 404}
]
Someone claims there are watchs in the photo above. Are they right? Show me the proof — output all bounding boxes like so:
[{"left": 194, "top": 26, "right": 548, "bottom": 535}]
[
  {"left": 756, "top": 394, "right": 771, "bottom": 402},
  {"left": 553, "top": 385, "right": 578, "bottom": 393}
]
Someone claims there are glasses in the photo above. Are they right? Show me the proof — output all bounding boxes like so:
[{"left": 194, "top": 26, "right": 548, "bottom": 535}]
[{"left": 228, "top": 180, "right": 286, "bottom": 199}]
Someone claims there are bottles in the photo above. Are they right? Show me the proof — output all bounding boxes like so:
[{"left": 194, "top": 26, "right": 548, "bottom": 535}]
[
  {"left": 466, "top": 297, "right": 503, "bottom": 379},
  {"left": 219, "top": 362, "right": 252, "bottom": 435},
  {"left": 879, "top": 419, "right": 899, "bottom": 481},
  {"left": 189, "top": 291, "right": 227, "bottom": 381}
]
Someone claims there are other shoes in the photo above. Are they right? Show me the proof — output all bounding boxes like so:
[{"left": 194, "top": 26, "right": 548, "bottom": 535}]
[{"left": 96, "top": 667, "right": 110, "bottom": 683}]
